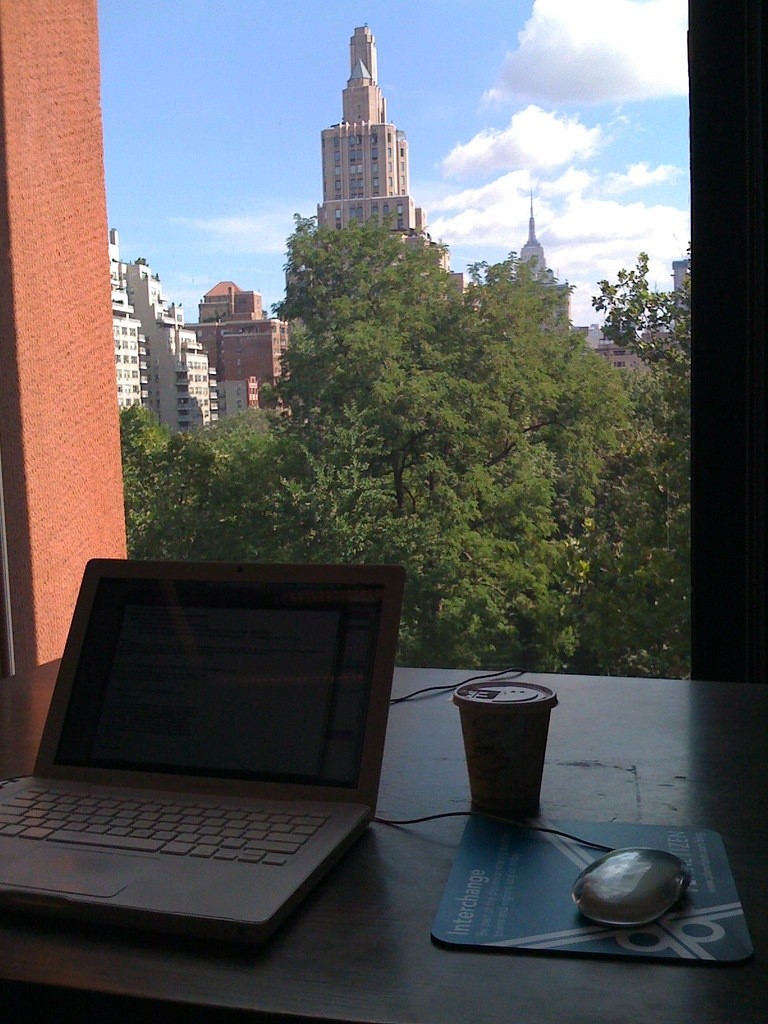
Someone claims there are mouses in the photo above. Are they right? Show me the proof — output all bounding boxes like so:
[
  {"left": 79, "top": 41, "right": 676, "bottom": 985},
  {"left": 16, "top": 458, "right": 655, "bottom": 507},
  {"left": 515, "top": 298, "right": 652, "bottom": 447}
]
[{"left": 573, "top": 846, "right": 691, "bottom": 926}]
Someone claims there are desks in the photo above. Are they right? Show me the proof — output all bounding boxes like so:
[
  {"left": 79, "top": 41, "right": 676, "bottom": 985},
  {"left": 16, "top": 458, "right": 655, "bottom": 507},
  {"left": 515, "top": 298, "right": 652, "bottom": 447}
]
[{"left": 1, "top": 656, "right": 767, "bottom": 1024}]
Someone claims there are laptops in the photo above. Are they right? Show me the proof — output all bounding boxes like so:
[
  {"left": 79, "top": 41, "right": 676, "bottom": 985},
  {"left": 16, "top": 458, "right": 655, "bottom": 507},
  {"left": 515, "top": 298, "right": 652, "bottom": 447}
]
[{"left": 1, "top": 560, "right": 409, "bottom": 945}]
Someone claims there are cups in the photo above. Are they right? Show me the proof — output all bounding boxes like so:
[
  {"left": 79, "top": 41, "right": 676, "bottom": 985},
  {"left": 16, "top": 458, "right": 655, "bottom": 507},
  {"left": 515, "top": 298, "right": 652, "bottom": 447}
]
[{"left": 451, "top": 681, "right": 558, "bottom": 815}]
